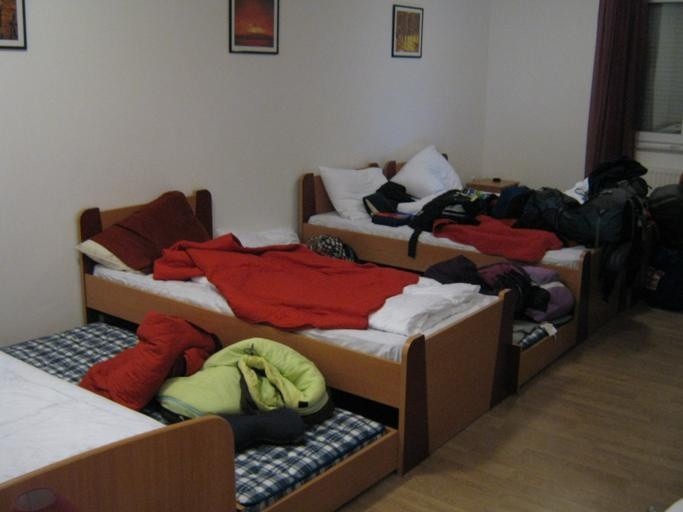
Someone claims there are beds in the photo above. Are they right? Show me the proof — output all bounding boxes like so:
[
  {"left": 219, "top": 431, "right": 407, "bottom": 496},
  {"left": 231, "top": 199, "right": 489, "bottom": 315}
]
[
  {"left": 298, "top": 154, "right": 654, "bottom": 389},
  {"left": 0, "top": 321, "right": 400, "bottom": 512},
  {"left": 77, "top": 154, "right": 513, "bottom": 476}
]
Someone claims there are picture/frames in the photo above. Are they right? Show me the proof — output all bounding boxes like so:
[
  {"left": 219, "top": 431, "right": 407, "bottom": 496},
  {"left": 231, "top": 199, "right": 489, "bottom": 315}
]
[
  {"left": 229, "top": 0, "right": 278, "bottom": 53},
  {"left": 393, "top": 5, "right": 423, "bottom": 57},
  {"left": 0, "top": 0, "right": 26, "bottom": 49}
]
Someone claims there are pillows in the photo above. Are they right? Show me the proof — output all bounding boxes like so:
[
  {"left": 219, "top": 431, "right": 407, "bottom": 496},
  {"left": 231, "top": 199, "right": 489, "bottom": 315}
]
[
  {"left": 389, "top": 144, "right": 463, "bottom": 198},
  {"left": 78, "top": 190, "right": 210, "bottom": 275}
]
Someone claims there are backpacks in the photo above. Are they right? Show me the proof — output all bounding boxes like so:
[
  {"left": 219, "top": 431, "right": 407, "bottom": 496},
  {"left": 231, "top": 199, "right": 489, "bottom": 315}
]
[{"left": 585, "top": 181, "right": 683, "bottom": 315}]
